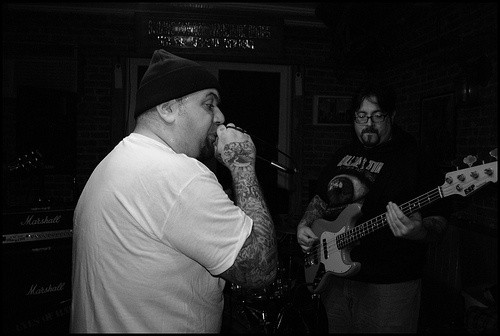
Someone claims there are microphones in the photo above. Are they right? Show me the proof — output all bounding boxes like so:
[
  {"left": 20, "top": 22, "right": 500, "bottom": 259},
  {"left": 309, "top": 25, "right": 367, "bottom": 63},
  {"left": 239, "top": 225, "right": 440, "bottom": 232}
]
[{"left": 206, "top": 130, "right": 293, "bottom": 177}]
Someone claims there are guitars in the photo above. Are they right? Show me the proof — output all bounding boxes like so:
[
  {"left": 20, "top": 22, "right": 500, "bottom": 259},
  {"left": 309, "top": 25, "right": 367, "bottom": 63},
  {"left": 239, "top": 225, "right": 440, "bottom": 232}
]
[{"left": 303, "top": 159, "right": 498, "bottom": 291}]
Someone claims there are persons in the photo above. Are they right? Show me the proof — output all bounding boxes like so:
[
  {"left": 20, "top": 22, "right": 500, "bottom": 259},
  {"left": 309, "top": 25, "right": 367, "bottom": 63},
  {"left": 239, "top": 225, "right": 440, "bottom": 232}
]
[
  {"left": 297, "top": 92, "right": 447, "bottom": 336},
  {"left": 63, "top": 49, "right": 278, "bottom": 334}
]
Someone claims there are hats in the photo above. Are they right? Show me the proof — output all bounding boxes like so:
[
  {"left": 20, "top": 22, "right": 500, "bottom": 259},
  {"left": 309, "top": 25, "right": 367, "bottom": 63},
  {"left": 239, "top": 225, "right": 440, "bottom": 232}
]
[{"left": 133, "top": 50, "right": 220, "bottom": 118}]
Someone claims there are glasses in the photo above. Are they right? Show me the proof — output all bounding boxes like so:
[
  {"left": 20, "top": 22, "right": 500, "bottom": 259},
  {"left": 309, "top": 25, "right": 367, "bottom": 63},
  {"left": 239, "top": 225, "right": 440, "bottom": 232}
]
[{"left": 355, "top": 113, "right": 387, "bottom": 124}]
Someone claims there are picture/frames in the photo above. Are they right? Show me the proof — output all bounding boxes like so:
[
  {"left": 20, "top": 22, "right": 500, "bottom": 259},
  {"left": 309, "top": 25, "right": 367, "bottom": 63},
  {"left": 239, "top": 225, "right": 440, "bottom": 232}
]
[
  {"left": 312, "top": 94, "right": 361, "bottom": 127},
  {"left": 419, "top": 92, "right": 455, "bottom": 135}
]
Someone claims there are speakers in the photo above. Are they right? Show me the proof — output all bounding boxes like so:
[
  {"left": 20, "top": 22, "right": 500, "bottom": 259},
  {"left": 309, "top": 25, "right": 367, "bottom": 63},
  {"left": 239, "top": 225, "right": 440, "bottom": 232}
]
[{"left": 0, "top": 204, "right": 74, "bottom": 313}]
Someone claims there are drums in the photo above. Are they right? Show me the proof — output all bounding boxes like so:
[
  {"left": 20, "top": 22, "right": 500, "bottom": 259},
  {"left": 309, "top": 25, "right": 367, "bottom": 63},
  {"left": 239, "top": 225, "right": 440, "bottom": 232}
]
[{"left": 270, "top": 215, "right": 303, "bottom": 256}]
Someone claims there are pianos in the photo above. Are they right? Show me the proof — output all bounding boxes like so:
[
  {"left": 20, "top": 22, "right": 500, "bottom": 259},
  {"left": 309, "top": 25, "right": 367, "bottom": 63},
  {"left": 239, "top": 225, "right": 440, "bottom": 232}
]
[{"left": 0, "top": 209, "right": 72, "bottom": 335}]
